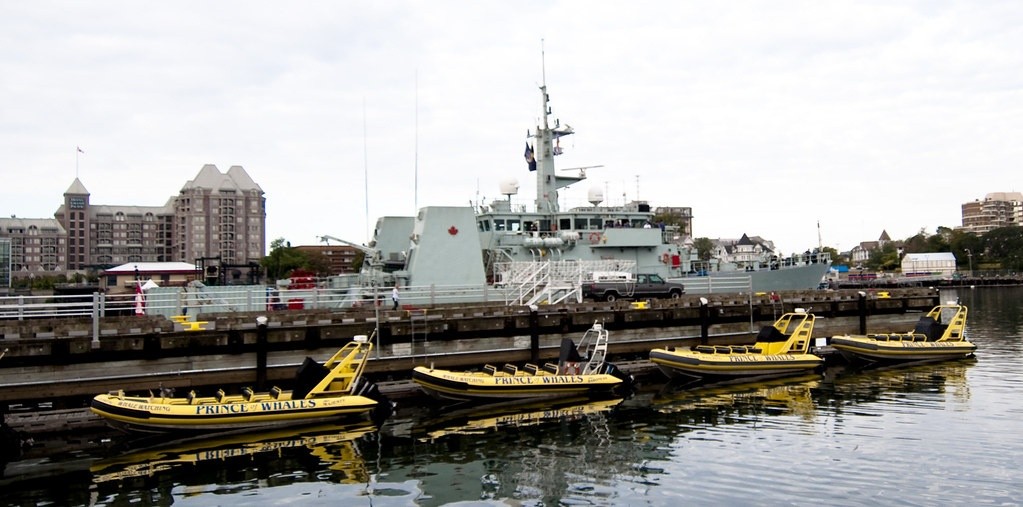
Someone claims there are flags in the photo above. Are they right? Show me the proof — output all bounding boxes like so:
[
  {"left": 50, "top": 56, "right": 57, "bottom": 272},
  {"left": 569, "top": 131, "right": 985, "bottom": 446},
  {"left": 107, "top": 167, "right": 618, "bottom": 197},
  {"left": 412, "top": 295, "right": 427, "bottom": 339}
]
[
  {"left": 524, "top": 142, "right": 536, "bottom": 171},
  {"left": 134, "top": 284, "right": 145, "bottom": 315}
]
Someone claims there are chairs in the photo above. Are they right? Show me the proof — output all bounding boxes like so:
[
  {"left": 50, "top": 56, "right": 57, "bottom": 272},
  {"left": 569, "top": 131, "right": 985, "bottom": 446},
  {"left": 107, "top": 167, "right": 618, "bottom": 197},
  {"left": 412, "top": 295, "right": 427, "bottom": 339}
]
[
  {"left": 864, "top": 332, "right": 927, "bottom": 343},
  {"left": 171, "top": 385, "right": 282, "bottom": 406},
  {"left": 479, "top": 363, "right": 560, "bottom": 378},
  {"left": 694, "top": 344, "right": 763, "bottom": 356}
]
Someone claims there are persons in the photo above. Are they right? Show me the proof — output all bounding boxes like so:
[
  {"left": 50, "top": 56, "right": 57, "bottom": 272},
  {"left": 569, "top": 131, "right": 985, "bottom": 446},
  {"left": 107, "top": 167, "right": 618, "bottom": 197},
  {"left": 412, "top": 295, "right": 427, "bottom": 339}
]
[
  {"left": 644, "top": 221, "right": 651, "bottom": 229},
  {"left": 529, "top": 222, "right": 537, "bottom": 235},
  {"left": 828, "top": 278, "right": 837, "bottom": 290},
  {"left": 604, "top": 220, "right": 621, "bottom": 228},
  {"left": 771, "top": 256, "right": 779, "bottom": 270},
  {"left": 659, "top": 220, "right": 665, "bottom": 231},
  {"left": 392, "top": 284, "right": 400, "bottom": 310},
  {"left": 624, "top": 221, "right": 630, "bottom": 227},
  {"left": 698, "top": 266, "right": 707, "bottom": 276}
]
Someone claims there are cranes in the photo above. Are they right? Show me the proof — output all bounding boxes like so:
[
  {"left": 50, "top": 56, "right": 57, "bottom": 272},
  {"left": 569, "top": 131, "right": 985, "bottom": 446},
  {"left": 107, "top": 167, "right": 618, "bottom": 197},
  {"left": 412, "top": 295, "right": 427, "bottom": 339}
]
[{"left": 321, "top": 235, "right": 384, "bottom": 286}]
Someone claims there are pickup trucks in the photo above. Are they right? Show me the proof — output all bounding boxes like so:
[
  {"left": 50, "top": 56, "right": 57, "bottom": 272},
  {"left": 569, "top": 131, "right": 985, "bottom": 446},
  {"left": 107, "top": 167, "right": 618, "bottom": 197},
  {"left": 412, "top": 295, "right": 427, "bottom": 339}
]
[{"left": 582, "top": 273, "right": 686, "bottom": 303}]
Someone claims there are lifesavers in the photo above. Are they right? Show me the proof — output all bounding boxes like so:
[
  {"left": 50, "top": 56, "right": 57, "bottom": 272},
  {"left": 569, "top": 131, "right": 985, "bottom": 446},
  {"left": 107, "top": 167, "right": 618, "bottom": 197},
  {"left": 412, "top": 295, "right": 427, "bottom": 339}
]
[
  {"left": 662, "top": 253, "right": 669, "bottom": 263},
  {"left": 589, "top": 232, "right": 601, "bottom": 246}
]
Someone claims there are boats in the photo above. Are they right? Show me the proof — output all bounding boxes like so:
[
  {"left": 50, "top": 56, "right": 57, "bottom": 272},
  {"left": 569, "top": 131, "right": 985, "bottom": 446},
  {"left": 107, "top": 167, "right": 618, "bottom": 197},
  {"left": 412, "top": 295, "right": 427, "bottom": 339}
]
[
  {"left": 652, "top": 374, "right": 821, "bottom": 414},
  {"left": 412, "top": 319, "right": 624, "bottom": 403},
  {"left": 835, "top": 355, "right": 979, "bottom": 400},
  {"left": 90, "top": 421, "right": 377, "bottom": 486},
  {"left": 650, "top": 307, "right": 825, "bottom": 382},
  {"left": 413, "top": 395, "right": 622, "bottom": 445},
  {"left": 135, "top": 37, "right": 833, "bottom": 312},
  {"left": 90, "top": 340, "right": 380, "bottom": 433},
  {"left": 830, "top": 299, "right": 977, "bottom": 363}
]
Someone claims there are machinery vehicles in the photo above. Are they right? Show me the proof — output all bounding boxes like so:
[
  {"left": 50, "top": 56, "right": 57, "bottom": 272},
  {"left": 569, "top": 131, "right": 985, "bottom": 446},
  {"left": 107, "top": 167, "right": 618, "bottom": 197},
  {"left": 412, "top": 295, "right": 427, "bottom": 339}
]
[
  {"left": 218, "top": 261, "right": 287, "bottom": 310},
  {"left": 195, "top": 255, "right": 222, "bottom": 284}
]
